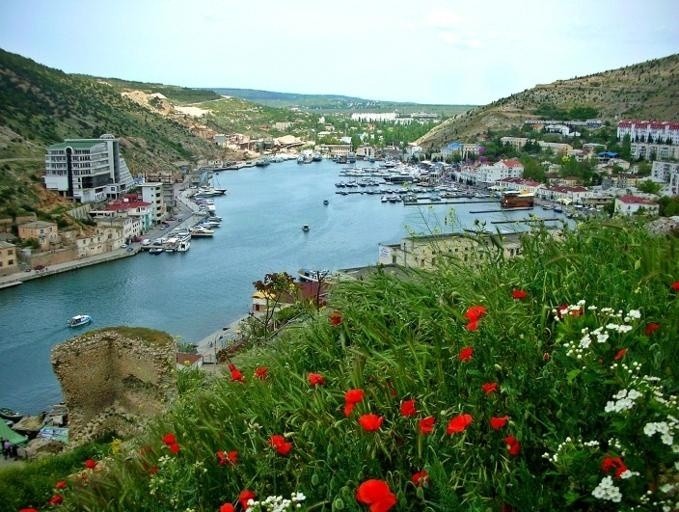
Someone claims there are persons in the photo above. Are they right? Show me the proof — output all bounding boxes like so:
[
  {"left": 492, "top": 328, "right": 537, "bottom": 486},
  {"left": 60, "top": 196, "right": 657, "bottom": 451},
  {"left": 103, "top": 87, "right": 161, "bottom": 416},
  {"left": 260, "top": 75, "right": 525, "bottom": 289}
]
[{"left": 4, "top": 440, "right": 12, "bottom": 460}]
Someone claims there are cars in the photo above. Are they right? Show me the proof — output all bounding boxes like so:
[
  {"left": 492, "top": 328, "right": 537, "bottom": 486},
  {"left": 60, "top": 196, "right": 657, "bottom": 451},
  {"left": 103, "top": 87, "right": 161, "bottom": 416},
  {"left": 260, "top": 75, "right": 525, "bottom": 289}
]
[
  {"left": 120, "top": 228, "right": 180, "bottom": 252},
  {"left": 34, "top": 265, "right": 45, "bottom": 270}
]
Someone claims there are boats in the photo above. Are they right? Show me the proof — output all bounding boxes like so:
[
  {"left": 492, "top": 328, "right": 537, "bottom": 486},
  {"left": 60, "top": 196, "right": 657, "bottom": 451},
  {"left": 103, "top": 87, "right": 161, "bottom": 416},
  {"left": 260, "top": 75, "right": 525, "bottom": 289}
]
[
  {"left": 303, "top": 224, "right": 309, "bottom": 232},
  {"left": 166, "top": 190, "right": 226, "bottom": 253},
  {"left": 543, "top": 205, "right": 562, "bottom": 213},
  {"left": 381, "top": 195, "right": 403, "bottom": 202},
  {"left": 323, "top": 200, "right": 329, "bottom": 205},
  {"left": 69, "top": 314, "right": 91, "bottom": 328},
  {"left": 333, "top": 156, "right": 355, "bottom": 163},
  {"left": 297, "top": 155, "right": 322, "bottom": 164},
  {"left": 336, "top": 167, "right": 462, "bottom": 195}
]
